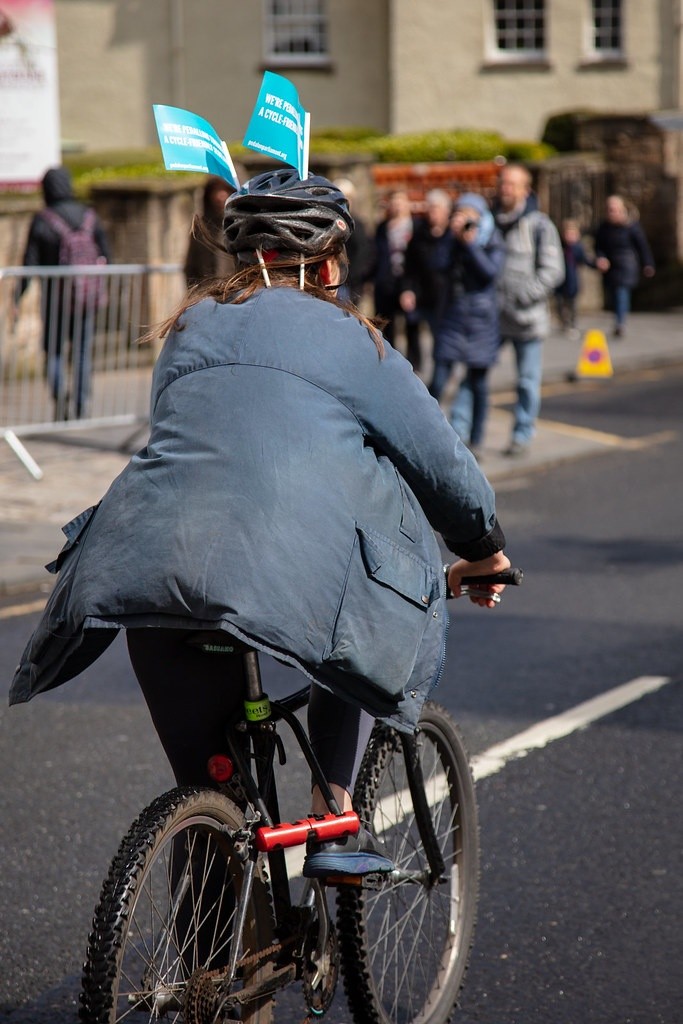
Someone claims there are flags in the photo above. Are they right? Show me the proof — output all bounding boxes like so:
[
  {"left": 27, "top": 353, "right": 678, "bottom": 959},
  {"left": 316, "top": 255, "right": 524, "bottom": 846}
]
[
  {"left": 152, "top": 103, "right": 237, "bottom": 189},
  {"left": 240, "top": 72, "right": 311, "bottom": 183}
]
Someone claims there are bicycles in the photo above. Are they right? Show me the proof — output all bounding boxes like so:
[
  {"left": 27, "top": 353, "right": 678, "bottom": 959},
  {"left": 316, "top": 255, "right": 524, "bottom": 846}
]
[{"left": 74, "top": 567, "right": 527, "bottom": 1023}]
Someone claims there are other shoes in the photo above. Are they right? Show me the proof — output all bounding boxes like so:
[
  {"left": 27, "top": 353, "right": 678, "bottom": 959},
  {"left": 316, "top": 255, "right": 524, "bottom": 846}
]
[
  {"left": 466, "top": 443, "right": 484, "bottom": 462},
  {"left": 504, "top": 440, "right": 530, "bottom": 456},
  {"left": 615, "top": 324, "right": 628, "bottom": 337},
  {"left": 52, "top": 390, "right": 71, "bottom": 421}
]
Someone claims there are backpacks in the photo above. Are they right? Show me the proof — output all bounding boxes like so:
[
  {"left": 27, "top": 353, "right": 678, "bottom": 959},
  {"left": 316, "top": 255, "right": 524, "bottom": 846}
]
[{"left": 34, "top": 205, "right": 110, "bottom": 315}]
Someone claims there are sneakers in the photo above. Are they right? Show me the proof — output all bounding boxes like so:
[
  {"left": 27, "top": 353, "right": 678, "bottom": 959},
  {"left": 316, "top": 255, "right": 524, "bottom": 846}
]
[{"left": 302, "top": 818, "right": 395, "bottom": 878}]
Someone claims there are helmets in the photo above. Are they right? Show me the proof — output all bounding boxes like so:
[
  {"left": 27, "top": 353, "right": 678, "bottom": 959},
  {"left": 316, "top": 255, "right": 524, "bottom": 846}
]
[{"left": 221, "top": 168, "right": 357, "bottom": 265}]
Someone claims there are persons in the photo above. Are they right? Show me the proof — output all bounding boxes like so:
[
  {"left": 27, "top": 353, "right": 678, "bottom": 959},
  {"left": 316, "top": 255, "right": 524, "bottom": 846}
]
[
  {"left": 554, "top": 195, "right": 654, "bottom": 336},
  {"left": 10, "top": 168, "right": 115, "bottom": 422},
  {"left": 9, "top": 172, "right": 511, "bottom": 1021},
  {"left": 450, "top": 167, "right": 570, "bottom": 459},
  {"left": 183, "top": 176, "right": 507, "bottom": 462}
]
